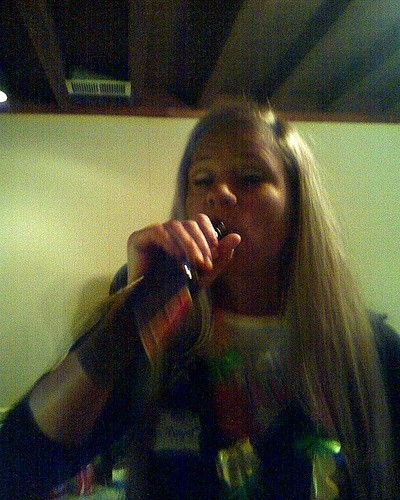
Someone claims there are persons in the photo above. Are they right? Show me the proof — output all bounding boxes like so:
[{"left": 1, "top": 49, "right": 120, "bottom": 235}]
[{"left": 1, "top": 104, "right": 398, "bottom": 500}]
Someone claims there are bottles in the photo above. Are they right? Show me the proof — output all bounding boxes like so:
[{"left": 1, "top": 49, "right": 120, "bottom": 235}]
[{"left": 91, "top": 219, "right": 232, "bottom": 379}]
